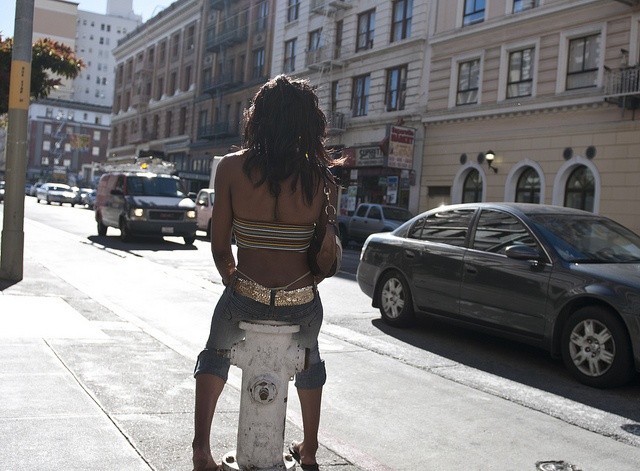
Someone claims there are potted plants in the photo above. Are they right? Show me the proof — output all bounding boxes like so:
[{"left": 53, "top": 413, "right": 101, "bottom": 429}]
[{"left": 0, "top": 35, "right": 86, "bottom": 116}]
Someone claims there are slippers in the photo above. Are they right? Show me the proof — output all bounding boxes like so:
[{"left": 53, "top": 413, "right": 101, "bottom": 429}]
[{"left": 289, "top": 440, "right": 319, "bottom": 469}]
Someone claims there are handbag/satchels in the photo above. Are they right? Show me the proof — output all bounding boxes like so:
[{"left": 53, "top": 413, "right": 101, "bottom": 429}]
[{"left": 308, "top": 185, "right": 342, "bottom": 278}]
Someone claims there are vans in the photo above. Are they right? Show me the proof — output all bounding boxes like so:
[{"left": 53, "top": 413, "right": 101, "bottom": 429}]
[{"left": 94, "top": 168, "right": 198, "bottom": 247}]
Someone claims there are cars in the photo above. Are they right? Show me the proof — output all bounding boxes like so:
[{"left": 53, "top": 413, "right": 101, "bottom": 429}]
[
  {"left": 80, "top": 187, "right": 96, "bottom": 208},
  {"left": 25, "top": 182, "right": 31, "bottom": 194},
  {"left": 0, "top": 181, "right": 6, "bottom": 202},
  {"left": 195, "top": 188, "right": 215, "bottom": 239},
  {"left": 354, "top": 201, "right": 639, "bottom": 378},
  {"left": 36, "top": 183, "right": 78, "bottom": 208},
  {"left": 31, "top": 181, "right": 42, "bottom": 196}
]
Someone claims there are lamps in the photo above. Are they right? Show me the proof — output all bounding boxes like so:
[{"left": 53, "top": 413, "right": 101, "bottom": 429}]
[{"left": 484, "top": 150, "right": 499, "bottom": 173}]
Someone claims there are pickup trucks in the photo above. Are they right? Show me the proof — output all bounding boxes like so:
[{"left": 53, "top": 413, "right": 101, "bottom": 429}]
[{"left": 336, "top": 203, "right": 414, "bottom": 243}]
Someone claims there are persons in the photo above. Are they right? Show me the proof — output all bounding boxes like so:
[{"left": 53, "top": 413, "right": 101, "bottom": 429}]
[{"left": 191, "top": 75, "right": 340, "bottom": 471}]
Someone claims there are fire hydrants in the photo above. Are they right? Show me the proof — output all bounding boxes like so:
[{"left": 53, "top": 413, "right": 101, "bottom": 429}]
[{"left": 223, "top": 319, "right": 306, "bottom": 470}]
